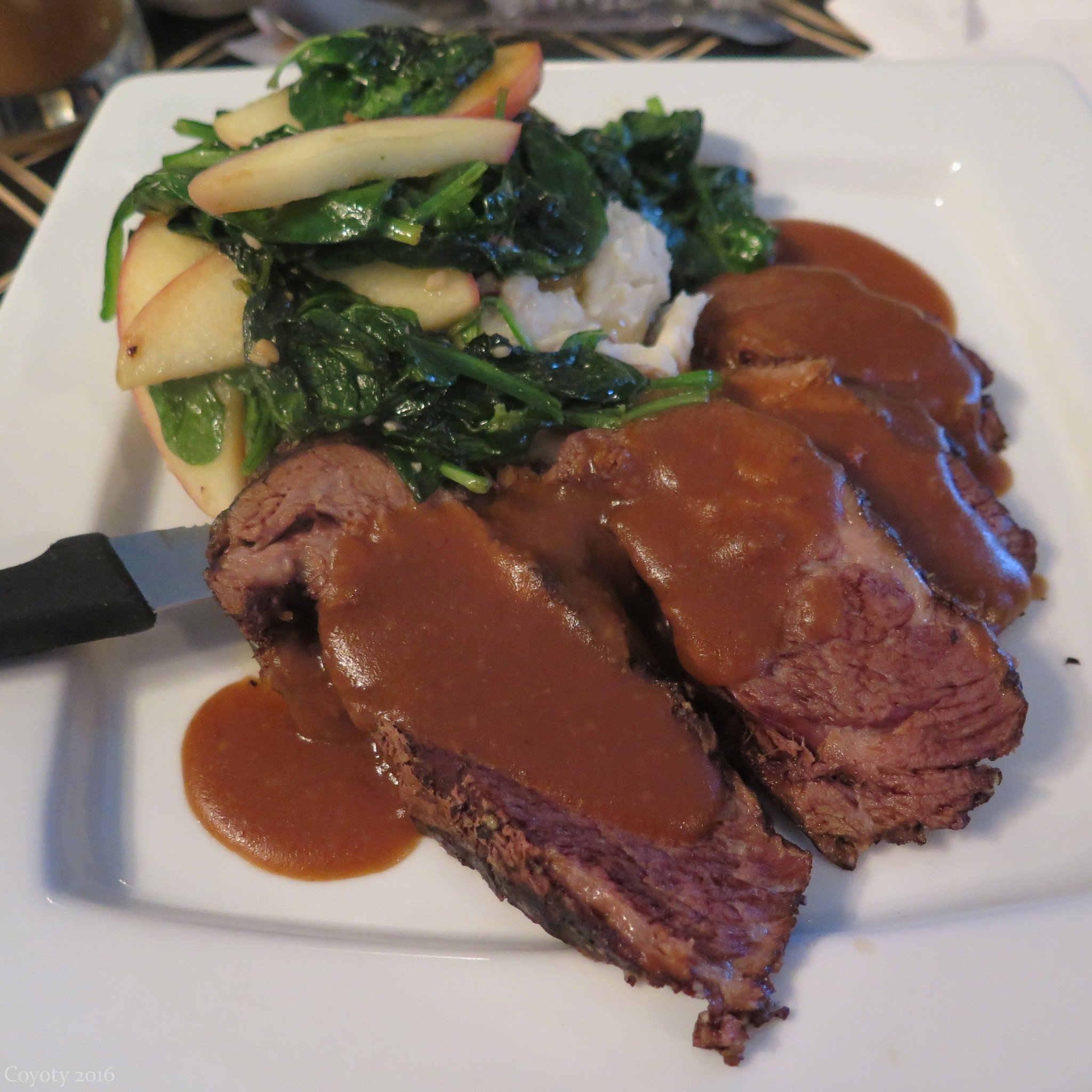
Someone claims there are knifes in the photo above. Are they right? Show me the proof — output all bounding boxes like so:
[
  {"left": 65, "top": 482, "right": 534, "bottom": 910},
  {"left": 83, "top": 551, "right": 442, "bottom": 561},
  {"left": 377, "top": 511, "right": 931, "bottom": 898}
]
[{"left": 0, "top": 523, "right": 216, "bottom": 668}]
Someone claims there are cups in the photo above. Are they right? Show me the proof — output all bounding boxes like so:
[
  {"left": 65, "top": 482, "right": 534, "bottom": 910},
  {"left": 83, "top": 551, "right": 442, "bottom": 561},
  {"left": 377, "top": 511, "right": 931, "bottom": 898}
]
[{"left": 0, "top": 0, "right": 160, "bottom": 134}]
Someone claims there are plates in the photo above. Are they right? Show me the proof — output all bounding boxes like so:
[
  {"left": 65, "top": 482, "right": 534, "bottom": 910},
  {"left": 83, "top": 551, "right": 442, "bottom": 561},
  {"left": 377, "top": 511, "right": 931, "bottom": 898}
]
[{"left": 0, "top": 59, "right": 1091, "bottom": 1092}]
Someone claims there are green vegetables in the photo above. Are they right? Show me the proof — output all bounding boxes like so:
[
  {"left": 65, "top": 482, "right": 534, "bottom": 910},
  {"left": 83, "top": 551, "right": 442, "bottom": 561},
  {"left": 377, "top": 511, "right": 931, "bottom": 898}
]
[{"left": 104, "top": 27, "right": 778, "bottom": 493}]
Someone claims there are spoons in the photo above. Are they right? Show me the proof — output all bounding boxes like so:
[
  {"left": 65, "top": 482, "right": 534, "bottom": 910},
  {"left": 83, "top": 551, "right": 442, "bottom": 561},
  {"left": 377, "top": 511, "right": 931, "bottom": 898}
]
[{"left": 262, "top": 1, "right": 793, "bottom": 45}]
